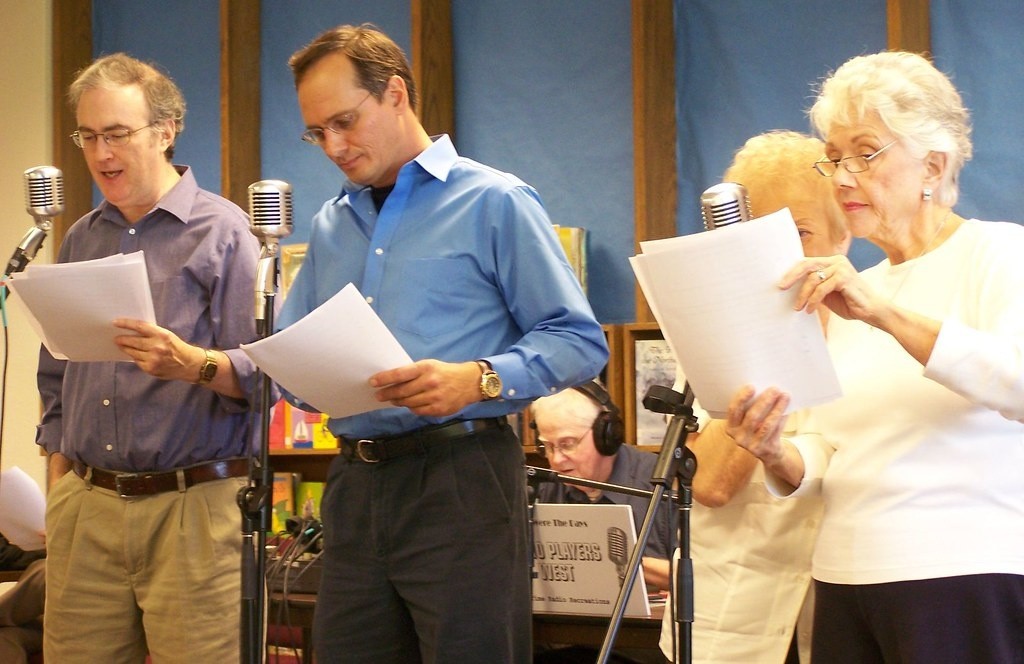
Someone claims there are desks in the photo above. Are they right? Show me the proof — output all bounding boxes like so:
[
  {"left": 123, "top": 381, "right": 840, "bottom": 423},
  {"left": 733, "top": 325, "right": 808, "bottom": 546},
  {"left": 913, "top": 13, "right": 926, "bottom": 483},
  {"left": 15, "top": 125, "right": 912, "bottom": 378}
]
[{"left": 266, "top": 590, "right": 670, "bottom": 664}]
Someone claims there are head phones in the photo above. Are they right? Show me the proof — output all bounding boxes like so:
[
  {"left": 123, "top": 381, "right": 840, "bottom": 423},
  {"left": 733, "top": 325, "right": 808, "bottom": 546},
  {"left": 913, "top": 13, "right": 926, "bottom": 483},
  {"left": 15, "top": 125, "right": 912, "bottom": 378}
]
[{"left": 529, "top": 379, "right": 626, "bottom": 456}]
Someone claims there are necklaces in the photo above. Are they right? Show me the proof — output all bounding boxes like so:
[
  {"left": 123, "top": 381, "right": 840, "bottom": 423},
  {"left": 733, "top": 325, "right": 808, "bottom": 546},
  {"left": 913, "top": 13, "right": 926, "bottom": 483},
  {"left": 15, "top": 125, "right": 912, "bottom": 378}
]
[{"left": 892, "top": 211, "right": 952, "bottom": 301}]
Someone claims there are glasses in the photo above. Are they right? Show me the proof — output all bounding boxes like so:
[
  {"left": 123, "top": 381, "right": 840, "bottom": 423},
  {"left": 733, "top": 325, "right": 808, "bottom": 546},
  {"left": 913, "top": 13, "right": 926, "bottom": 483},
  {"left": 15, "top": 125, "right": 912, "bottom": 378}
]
[
  {"left": 534, "top": 429, "right": 593, "bottom": 458},
  {"left": 812, "top": 140, "right": 897, "bottom": 178},
  {"left": 301, "top": 93, "right": 372, "bottom": 145},
  {"left": 69, "top": 122, "right": 156, "bottom": 149}
]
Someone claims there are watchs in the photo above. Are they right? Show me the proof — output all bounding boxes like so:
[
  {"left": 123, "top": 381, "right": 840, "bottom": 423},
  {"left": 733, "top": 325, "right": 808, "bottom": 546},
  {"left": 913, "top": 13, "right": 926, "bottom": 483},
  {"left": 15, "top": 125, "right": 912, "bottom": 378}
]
[
  {"left": 476, "top": 361, "right": 503, "bottom": 403},
  {"left": 191, "top": 348, "right": 218, "bottom": 387}
]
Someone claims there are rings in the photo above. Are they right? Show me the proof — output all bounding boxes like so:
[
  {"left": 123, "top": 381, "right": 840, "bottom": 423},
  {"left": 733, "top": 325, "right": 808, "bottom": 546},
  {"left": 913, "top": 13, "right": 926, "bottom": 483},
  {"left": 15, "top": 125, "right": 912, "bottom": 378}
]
[{"left": 817, "top": 271, "right": 827, "bottom": 281}]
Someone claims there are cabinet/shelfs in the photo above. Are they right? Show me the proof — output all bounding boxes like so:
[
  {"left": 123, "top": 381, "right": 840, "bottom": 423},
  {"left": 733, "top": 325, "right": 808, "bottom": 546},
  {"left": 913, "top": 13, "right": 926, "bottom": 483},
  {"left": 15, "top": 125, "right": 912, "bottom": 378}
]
[{"left": 265, "top": 322, "right": 710, "bottom": 554}]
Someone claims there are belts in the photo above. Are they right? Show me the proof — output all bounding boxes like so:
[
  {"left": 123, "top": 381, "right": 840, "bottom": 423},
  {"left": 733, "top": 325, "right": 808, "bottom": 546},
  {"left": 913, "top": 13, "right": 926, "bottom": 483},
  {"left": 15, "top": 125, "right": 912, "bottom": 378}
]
[
  {"left": 72, "top": 457, "right": 249, "bottom": 499},
  {"left": 338, "top": 415, "right": 508, "bottom": 464}
]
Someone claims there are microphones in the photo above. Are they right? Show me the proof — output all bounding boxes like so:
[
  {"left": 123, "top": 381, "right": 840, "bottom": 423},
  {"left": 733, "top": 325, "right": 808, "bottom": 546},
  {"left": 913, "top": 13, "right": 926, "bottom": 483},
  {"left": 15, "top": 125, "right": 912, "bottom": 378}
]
[
  {"left": 700, "top": 182, "right": 754, "bottom": 232},
  {"left": 248, "top": 178, "right": 295, "bottom": 337},
  {"left": 2, "top": 165, "right": 67, "bottom": 298},
  {"left": 607, "top": 528, "right": 628, "bottom": 588}
]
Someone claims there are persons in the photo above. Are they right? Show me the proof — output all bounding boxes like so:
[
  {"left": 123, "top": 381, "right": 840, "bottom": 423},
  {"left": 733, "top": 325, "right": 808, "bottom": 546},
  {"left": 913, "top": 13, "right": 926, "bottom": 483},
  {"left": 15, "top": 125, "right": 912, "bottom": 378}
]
[
  {"left": 721, "top": 48, "right": 1023, "bottom": 664},
  {"left": 34, "top": 50, "right": 258, "bottom": 663},
  {"left": 659, "top": 130, "right": 852, "bottom": 664},
  {"left": 523, "top": 382, "right": 675, "bottom": 596},
  {"left": 271, "top": 21, "right": 613, "bottom": 664},
  {"left": 0, "top": 529, "right": 45, "bottom": 664}
]
